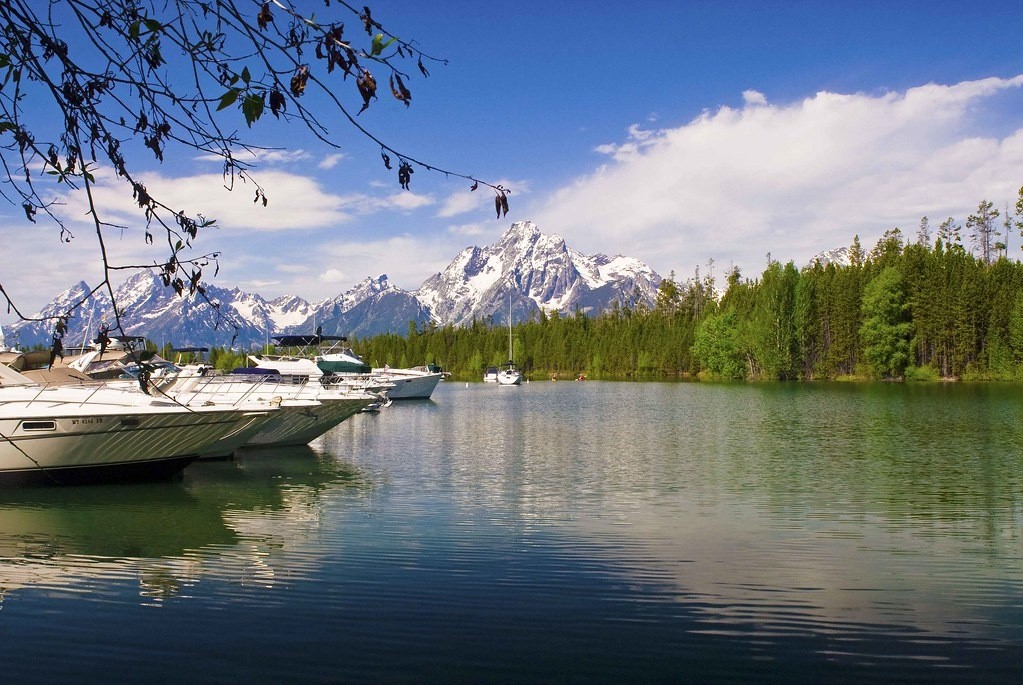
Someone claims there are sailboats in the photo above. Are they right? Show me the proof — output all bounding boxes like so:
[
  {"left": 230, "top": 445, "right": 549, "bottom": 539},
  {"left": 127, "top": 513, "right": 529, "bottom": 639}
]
[{"left": 482, "top": 295, "right": 523, "bottom": 384}]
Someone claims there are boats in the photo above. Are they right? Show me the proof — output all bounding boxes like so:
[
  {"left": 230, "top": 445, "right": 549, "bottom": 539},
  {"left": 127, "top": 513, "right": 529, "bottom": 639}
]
[{"left": 0, "top": 291, "right": 452, "bottom": 485}]
